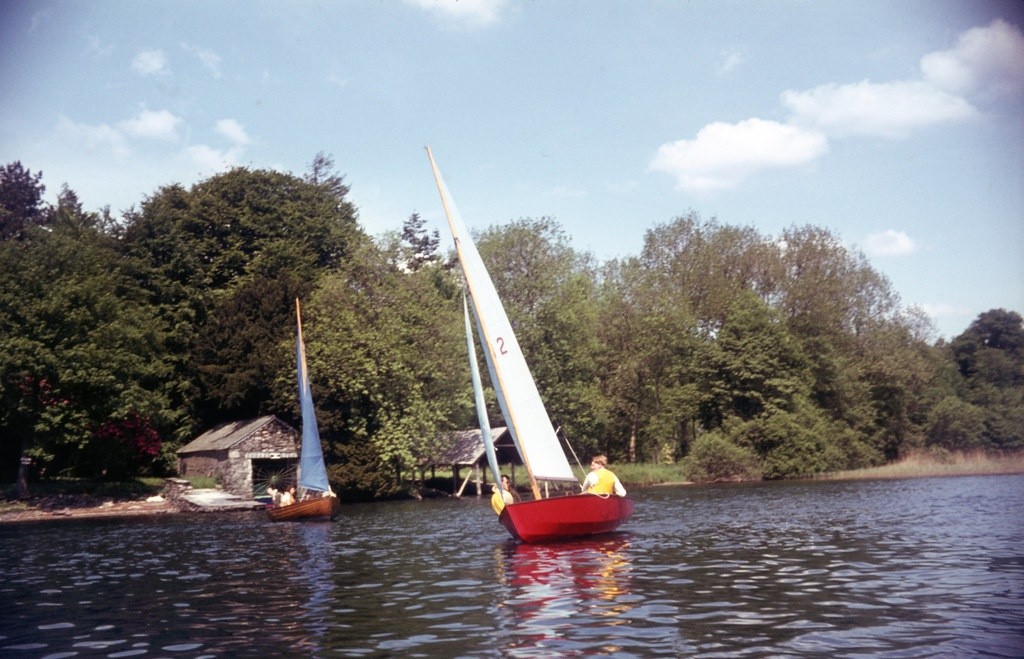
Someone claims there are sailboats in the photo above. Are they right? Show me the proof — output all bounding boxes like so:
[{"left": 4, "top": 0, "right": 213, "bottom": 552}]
[
  {"left": 427, "top": 146, "right": 634, "bottom": 543},
  {"left": 266, "top": 297, "right": 340, "bottom": 521}
]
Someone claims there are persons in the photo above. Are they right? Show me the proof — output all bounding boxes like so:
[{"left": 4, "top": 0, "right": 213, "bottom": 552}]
[
  {"left": 491, "top": 474, "right": 521, "bottom": 516},
  {"left": 290, "top": 485, "right": 298, "bottom": 502},
  {"left": 279, "top": 485, "right": 297, "bottom": 506},
  {"left": 579, "top": 454, "right": 627, "bottom": 500},
  {"left": 274, "top": 488, "right": 286, "bottom": 506}
]
